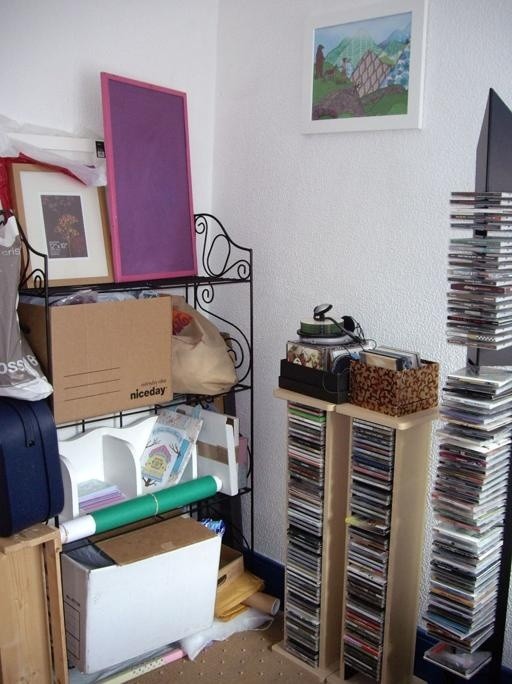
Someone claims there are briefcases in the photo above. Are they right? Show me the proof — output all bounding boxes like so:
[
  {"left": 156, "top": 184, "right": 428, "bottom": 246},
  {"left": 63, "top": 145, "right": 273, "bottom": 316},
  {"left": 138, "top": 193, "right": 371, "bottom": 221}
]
[{"left": 0, "top": 399, "right": 64, "bottom": 536}]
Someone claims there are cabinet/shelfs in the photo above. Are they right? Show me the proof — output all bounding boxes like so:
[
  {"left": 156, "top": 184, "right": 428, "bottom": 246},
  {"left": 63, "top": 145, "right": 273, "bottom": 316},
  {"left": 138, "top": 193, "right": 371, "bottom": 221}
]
[
  {"left": 271, "top": 388, "right": 442, "bottom": 684},
  {"left": 0, "top": 206, "right": 257, "bottom": 684}
]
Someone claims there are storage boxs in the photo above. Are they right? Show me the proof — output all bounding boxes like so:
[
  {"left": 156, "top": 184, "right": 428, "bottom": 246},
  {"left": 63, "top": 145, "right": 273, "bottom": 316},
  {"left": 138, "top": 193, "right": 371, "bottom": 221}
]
[
  {"left": 0, "top": 521, "right": 70, "bottom": 684},
  {"left": 16, "top": 294, "right": 173, "bottom": 428},
  {"left": 348, "top": 360, "right": 439, "bottom": 417},
  {"left": 61, "top": 509, "right": 223, "bottom": 674},
  {"left": 285, "top": 339, "right": 372, "bottom": 373},
  {"left": 217, "top": 540, "right": 245, "bottom": 593},
  {"left": 278, "top": 359, "right": 348, "bottom": 404}
]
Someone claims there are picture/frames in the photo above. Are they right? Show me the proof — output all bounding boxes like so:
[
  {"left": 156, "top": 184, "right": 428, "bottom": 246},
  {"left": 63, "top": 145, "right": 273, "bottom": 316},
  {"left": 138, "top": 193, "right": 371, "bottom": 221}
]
[
  {"left": 9, "top": 163, "right": 114, "bottom": 291},
  {"left": 303, "top": 0, "right": 428, "bottom": 134},
  {"left": 100, "top": 71, "right": 198, "bottom": 284},
  {"left": 4, "top": 133, "right": 107, "bottom": 182}
]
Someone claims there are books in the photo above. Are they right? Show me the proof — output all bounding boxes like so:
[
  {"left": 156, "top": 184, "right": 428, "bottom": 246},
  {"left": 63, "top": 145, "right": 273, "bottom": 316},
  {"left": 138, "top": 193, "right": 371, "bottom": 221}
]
[
  {"left": 136, "top": 407, "right": 204, "bottom": 489},
  {"left": 70, "top": 477, "right": 130, "bottom": 514}
]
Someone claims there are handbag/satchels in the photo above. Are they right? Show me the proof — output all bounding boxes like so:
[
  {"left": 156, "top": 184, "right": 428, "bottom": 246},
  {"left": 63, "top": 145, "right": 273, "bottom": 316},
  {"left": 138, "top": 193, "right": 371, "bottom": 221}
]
[{"left": 0, "top": 208, "right": 54, "bottom": 401}]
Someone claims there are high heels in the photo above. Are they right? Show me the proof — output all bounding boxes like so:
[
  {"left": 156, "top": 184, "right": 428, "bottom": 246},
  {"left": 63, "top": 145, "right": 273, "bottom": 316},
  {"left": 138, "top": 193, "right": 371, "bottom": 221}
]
[{"left": 161, "top": 291, "right": 238, "bottom": 401}]
